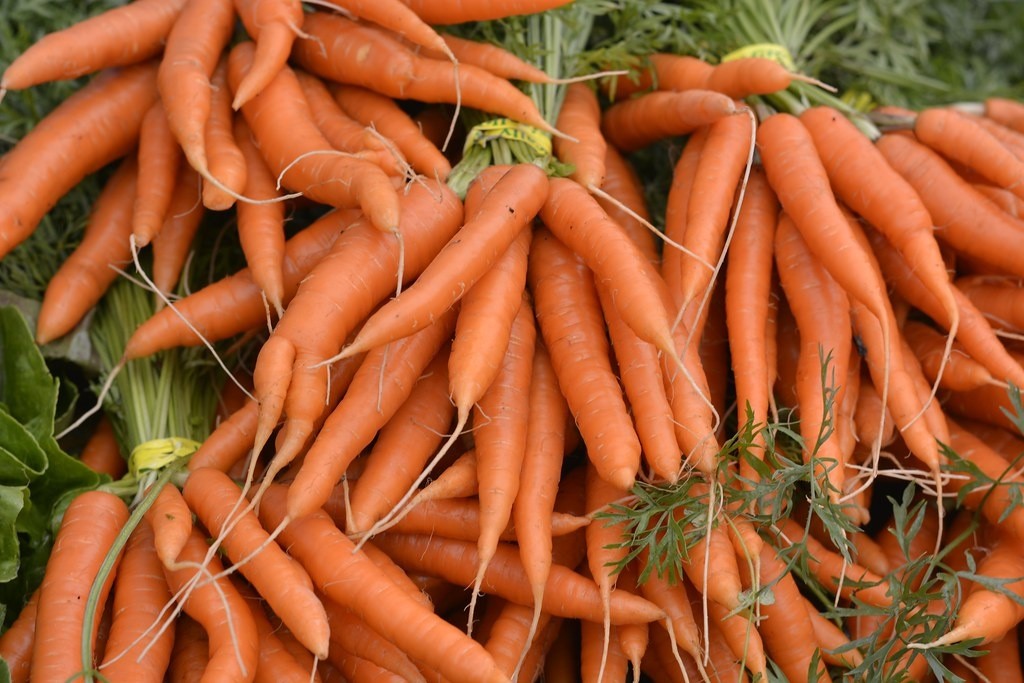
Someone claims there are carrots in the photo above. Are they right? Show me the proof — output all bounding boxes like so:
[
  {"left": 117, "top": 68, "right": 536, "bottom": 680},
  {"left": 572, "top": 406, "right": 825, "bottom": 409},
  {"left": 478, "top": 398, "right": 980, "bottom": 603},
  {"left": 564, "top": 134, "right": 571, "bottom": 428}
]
[{"left": 0, "top": 0, "right": 1024, "bottom": 683}]
